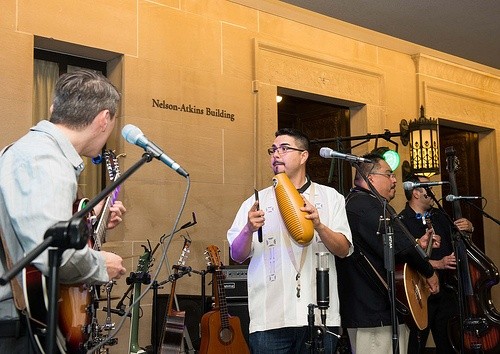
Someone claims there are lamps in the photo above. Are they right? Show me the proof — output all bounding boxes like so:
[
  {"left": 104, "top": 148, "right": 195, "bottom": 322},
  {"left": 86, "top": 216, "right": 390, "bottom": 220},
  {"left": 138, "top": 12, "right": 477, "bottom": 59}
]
[{"left": 408, "top": 105, "right": 440, "bottom": 177}]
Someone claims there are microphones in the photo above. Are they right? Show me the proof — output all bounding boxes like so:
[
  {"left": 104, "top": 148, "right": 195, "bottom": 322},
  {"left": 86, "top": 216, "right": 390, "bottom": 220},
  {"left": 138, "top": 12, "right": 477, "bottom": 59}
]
[
  {"left": 402, "top": 181, "right": 449, "bottom": 190},
  {"left": 320, "top": 147, "right": 372, "bottom": 163},
  {"left": 121, "top": 124, "right": 189, "bottom": 177},
  {"left": 445, "top": 194, "right": 483, "bottom": 202}
]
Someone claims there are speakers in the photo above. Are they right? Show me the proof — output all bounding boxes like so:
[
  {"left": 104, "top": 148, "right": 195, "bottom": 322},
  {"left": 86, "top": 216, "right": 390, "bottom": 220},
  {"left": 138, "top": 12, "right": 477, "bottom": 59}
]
[
  {"left": 150, "top": 294, "right": 214, "bottom": 354},
  {"left": 225, "top": 303, "right": 250, "bottom": 345}
]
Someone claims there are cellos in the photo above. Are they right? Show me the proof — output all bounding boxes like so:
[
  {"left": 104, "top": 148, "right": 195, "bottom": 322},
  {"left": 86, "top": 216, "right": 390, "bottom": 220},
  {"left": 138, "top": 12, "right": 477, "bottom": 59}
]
[{"left": 440, "top": 144, "right": 500, "bottom": 353}]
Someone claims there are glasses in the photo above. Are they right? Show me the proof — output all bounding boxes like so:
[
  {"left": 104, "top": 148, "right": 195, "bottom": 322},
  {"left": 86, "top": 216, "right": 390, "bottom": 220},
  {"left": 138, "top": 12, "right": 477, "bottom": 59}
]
[
  {"left": 267, "top": 147, "right": 305, "bottom": 155},
  {"left": 367, "top": 172, "right": 396, "bottom": 179}
]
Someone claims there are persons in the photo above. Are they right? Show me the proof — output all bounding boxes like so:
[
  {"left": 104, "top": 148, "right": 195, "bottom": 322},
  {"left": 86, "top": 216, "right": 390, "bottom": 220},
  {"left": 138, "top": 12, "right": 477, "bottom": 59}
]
[
  {"left": 227, "top": 130, "right": 354, "bottom": 354},
  {"left": 0, "top": 71, "right": 128, "bottom": 354},
  {"left": 335, "top": 154, "right": 440, "bottom": 354},
  {"left": 396, "top": 175, "right": 473, "bottom": 354}
]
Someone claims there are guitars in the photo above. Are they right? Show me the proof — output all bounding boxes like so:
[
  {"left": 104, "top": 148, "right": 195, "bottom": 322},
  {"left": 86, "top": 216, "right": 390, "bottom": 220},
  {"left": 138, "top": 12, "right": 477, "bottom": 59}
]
[
  {"left": 394, "top": 218, "right": 435, "bottom": 331},
  {"left": 127, "top": 239, "right": 187, "bottom": 354},
  {"left": 22, "top": 150, "right": 121, "bottom": 354},
  {"left": 198, "top": 245, "right": 250, "bottom": 354}
]
